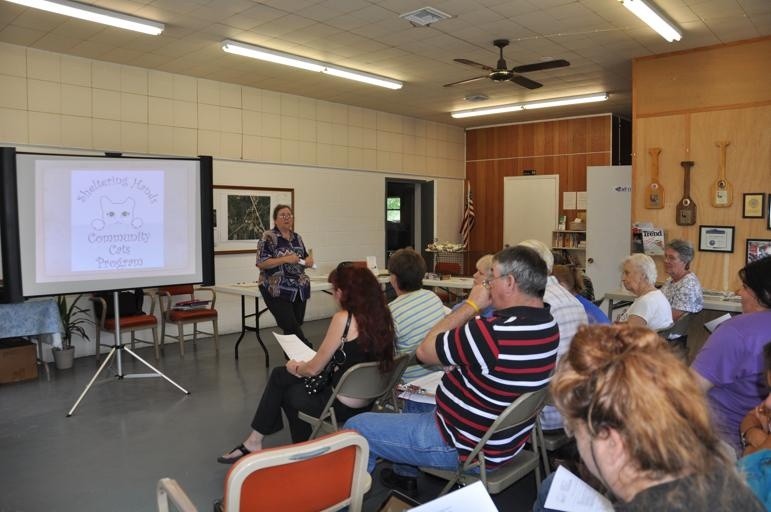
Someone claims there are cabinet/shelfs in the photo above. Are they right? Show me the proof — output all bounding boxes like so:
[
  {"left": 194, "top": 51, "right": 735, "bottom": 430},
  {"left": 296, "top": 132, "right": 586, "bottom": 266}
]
[{"left": 552, "top": 230, "right": 585, "bottom": 272}]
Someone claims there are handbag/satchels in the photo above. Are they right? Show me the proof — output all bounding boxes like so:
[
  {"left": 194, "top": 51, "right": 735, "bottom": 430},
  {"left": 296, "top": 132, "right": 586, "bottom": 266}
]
[{"left": 303, "top": 351, "right": 347, "bottom": 395}]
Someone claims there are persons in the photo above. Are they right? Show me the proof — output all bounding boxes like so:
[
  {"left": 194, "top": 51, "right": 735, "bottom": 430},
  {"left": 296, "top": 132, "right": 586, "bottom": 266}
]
[
  {"left": 688, "top": 252, "right": 771, "bottom": 455},
  {"left": 381, "top": 249, "right": 449, "bottom": 351},
  {"left": 549, "top": 323, "right": 771, "bottom": 511},
  {"left": 213, "top": 257, "right": 397, "bottom": 468},
  {"left": 256, "top": 202, "right": 314, "bottom": 362},
  {"left": 613, "top": 251, "right": 675, "bottom": 336},
  {"left": 345, "top": 241, "right": 612, "bottom": 502},
  {"left": 655, "top": 242, "right": 706, "bottom": 316}
]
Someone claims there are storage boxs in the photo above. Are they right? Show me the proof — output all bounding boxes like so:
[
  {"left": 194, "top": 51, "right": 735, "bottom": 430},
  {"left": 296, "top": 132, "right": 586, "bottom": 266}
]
[{"left": 0, "top": 338, "right": 37, "bottom": 383}]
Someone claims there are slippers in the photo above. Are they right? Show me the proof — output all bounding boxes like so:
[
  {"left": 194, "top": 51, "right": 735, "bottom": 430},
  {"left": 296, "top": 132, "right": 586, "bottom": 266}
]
[{"left": 218, "top": 444, "right": 248, "bottom": 464}]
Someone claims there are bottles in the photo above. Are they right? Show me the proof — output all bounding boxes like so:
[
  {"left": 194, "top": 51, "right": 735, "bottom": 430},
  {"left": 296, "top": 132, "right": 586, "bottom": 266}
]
[{"left": 559, "top": 233, "right": 578, "bottom": 248}]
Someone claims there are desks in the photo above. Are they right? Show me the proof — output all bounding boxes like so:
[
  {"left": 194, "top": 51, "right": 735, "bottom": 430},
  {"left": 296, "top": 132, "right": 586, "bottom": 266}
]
[
  {"left": 422, "top": 277, "right": 474, "bottom": 302},
  {"left": 0, "top": 297, "right": 61, "bottom": 383},
  {"left": 215, "top": 273, "right": 391, "bottom": 367},
  {"left": 605, "top": 287, "right": 743, "bottom": 323}
]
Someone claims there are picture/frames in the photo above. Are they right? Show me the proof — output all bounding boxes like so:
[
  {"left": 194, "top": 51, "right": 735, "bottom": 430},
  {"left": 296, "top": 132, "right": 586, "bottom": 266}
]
[
  {"left": 767, "top": 194, "right": 771, "bottom": 229},
  {"left": 746, "top": 239, "right": 771, "bottom": 264},
  {"left": 743, "top": 193, "right": 764, "bottom": 218},
  {"left": 698, "top": 225, "right": 735, "bottom": 253},
  {"left": 213, "top": 185, "right": 294, "bottom": 255}
]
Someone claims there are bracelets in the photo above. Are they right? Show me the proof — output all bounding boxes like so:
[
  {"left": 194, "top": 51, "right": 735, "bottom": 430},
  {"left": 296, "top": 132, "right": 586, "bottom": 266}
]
[{"left": 460, "top": 296, "right": 481, "bottom": 315}]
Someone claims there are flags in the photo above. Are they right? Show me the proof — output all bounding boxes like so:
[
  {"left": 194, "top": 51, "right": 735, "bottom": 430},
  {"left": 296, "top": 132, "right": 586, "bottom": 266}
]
[{"left": 459, "top": 184, "right": 477, "bottom": 249}]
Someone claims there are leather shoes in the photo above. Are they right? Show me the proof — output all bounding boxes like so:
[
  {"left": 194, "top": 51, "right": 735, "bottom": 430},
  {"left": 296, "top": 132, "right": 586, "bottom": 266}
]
[{"left": 379, "top": 468, "right": 418, "bottom": 496}]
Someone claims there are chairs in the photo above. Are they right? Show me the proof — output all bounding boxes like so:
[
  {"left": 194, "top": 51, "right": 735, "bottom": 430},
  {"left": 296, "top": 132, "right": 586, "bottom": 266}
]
[
  {"left": 435, "top": 262, "right": 462, "bottom": 302},
  {"left": 537, "top": 397, "right": 572, "bottom": 451},
  {"left": 299, "top": 352, "right": 409, "bottom": 440},
  {"left": 90, "top": 289, "right": 159, "bottom": 362},
  {"left": 592, "top": 297, "right": 606, "bottom": 308},
  {"left": 656, "top": 312, "right": 690, "bottom": 344},
  {"left": 156, "top": 430, "right": 372, "bottom": 511},
  {"left": 156, "top": 284, "right": 219, "bottom": 357},
  {"left": 420, "top": 390, "right": 551, "bottom": 497}
]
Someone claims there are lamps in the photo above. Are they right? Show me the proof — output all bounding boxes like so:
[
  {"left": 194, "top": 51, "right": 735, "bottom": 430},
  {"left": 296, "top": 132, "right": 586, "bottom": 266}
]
[
  {"left": 450, "top": 92, "right": 609, "bottom": 118},
  {"left": 622, "top": 0, "right": 682, "bottom": 43},
  {"left": 6, "top": 0, "right": 165, "bottom": 36},
  {"left": 221, "top": 38, "right": 404, "bottom": 90}
]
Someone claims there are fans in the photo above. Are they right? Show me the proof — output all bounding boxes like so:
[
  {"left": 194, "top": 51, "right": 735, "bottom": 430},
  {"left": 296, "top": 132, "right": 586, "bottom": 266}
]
[{"left": 443, "top": 39, "right": 571, "bottom": 89}]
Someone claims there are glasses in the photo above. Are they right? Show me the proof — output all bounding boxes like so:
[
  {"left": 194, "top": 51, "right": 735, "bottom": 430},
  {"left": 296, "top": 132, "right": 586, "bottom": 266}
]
[
  {"left": 279, "top": 213, "right": 294, "bottom": 220},
  {"left": 483, "top": 274, "right": 508, "bottom": 289}
]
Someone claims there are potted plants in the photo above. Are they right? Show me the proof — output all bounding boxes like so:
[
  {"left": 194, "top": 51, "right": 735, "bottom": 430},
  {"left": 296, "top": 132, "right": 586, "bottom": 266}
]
[{"left": 52, "top": 295, "right": 96, "bottom": 369}]
